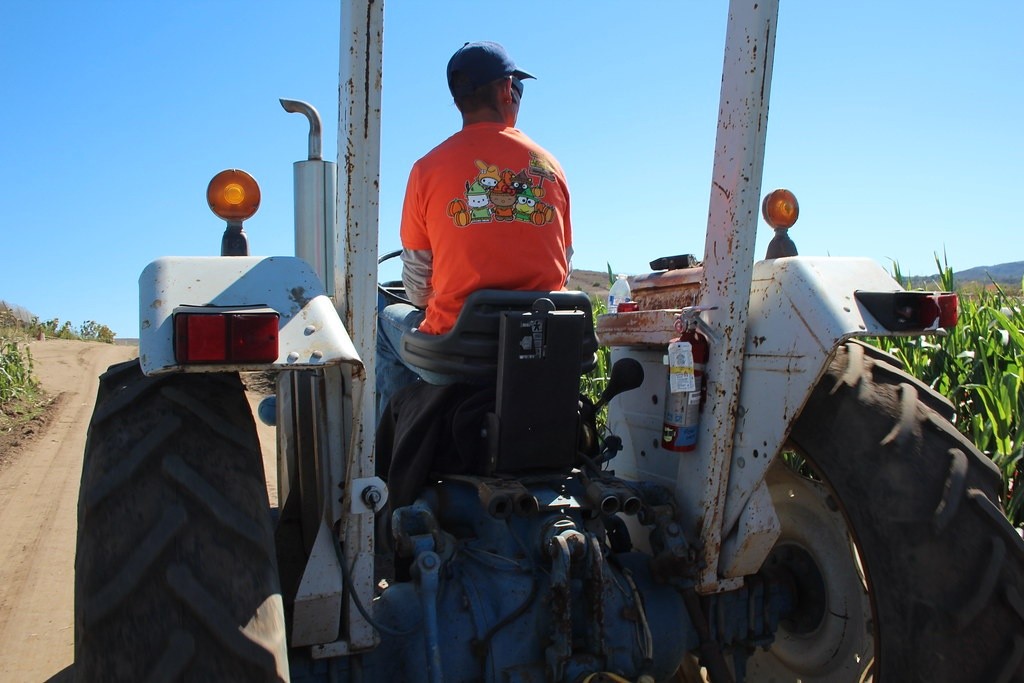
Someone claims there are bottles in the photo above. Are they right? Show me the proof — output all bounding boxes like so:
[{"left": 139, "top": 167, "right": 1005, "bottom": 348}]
[{"left": 607, "top": 275, "right": 632, "bottom": 312}]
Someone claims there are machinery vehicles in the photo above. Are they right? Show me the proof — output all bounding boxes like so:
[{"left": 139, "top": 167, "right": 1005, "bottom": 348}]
[{"left": 73, "top": 0, "right": 1024, "bottom": 683}]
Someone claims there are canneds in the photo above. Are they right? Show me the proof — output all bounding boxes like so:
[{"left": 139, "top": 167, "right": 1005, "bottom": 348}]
[{"left": 617, "top": 300, "right": 639, "bottom": 312}]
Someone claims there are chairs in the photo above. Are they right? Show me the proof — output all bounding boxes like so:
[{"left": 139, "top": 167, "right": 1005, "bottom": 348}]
[{"left": 398, "top": 291, "right": 600, "bottom": 474}]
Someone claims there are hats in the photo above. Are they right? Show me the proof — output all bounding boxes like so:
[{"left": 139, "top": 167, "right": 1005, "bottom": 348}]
[{"left": 447, "top": 42, "right": 538, "bottom": 96}]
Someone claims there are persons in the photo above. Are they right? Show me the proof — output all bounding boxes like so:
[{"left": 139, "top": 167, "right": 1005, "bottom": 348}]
[{"left": 377, "top": 41, "right": 575, "bottom": 419}]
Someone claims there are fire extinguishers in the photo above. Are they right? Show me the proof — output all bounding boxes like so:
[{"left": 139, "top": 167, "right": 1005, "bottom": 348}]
[{"left": 660, "top": 303, "right": 721, "bottom": 452}]
